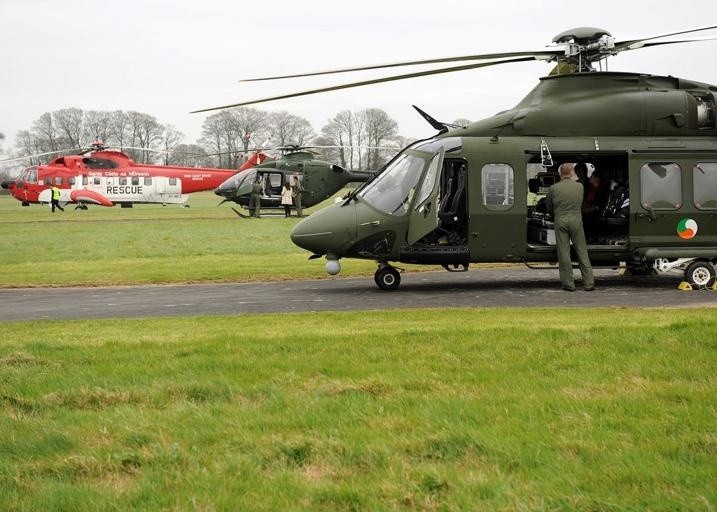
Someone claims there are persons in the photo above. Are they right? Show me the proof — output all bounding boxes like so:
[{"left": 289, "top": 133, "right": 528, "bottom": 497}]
[
  {"left": 546, "top": 161, "right": 595, "bottom": 292},
  {"left": 280, "top": 181, "right": 293, "bottom": 218},
  {"left": 48, "top": 183, "right": 64, "bottom": 213},
  {"left": 248, "top": 179, "right": 263, "bottom": 219},
  {"left": 293, "top": 175, "right": 304, "bottom": 218},
  {"left": 582, "top": 168, "right": 610, "bottom": 234}
]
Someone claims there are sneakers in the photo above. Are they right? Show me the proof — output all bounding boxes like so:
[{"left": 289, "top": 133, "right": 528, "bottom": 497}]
[{"left": 559, "top": 284, "right": 595, "bottom": 291}]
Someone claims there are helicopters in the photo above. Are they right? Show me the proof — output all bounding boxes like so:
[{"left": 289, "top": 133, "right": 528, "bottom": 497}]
[
  {"left": 0, "top": 134, "right": 268, "bottom": 210},
  {"left": 183, "top": 144, "right": 377, "bottom": 218},
  {"left": 189, "top": 26, "right": 717, "bottom": 291}
]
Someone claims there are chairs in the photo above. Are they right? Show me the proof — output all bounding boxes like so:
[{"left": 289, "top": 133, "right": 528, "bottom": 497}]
[
  {"left": 596, "top": 179, "right": 629, "bottom": 246},
  {"left": 438, "top": 164, "right": 466, "bottom": 245}
]
